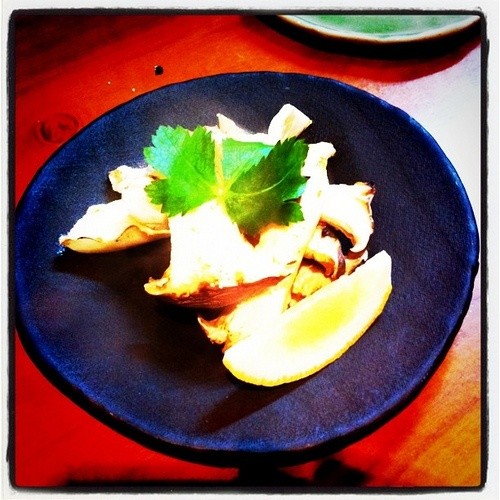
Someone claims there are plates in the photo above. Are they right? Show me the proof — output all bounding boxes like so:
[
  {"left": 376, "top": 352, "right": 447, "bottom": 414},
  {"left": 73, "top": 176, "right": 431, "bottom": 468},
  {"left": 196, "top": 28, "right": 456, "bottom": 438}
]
[
  {"left": 16, "top": 69, "right": 483, "bottom": 456},
  {"left": 280, "top": 16, "right": 477, "bottom": 47}
]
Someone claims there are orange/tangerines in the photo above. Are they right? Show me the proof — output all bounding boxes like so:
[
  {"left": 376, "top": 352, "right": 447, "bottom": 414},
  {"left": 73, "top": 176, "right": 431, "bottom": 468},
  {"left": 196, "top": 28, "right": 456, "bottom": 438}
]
[{"left": 221, "top": 250, "right": 392, "bottom": 387}]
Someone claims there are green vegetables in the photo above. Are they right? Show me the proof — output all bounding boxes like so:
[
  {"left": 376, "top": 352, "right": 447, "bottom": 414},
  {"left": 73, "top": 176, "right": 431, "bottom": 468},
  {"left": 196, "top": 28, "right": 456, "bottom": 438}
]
[{"left": 143, "top": 124, "right": 312, "bottom": 249}]
